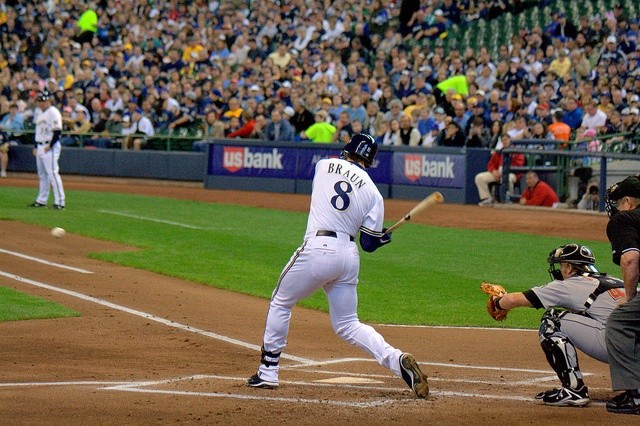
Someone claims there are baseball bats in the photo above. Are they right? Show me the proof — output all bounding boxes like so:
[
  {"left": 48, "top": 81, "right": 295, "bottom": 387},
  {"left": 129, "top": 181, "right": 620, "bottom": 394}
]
[
  {"left": 385, "top": 191, "right": 444, "bottom": 235},
  {"left": 553, "top": 203, "right": 578, "bottom": 209}
]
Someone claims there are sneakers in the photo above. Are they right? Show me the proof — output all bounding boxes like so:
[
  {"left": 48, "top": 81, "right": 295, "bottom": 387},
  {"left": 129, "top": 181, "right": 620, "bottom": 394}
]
[
  {"left": 400, "top": 353, "right": 429, "bottom": 399},
  {"left": 27, "top": 202, "right": 46, "bottom": 209},
  {"left": 543, "top": 386, "right": 589, "bottom": 408},
  {"left": 246, "top": 373, "right": 279, "bottom": 389},
  {"left": 478, "top": 200, "right": 495, "bottom": 206},
  {"left": 52, "top": 204, "right": 66, "bottom": 211},
  {"left": 606, "top": 392, "right": 639, "bottom": 414}
]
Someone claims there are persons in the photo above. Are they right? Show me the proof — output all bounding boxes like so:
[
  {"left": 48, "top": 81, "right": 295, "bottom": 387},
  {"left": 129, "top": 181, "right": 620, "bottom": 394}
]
[
  {"left": 66, "top": 1, "right": 639, "bottom": 150},
  {"left": 575, "top": 177, "right": 607, "bottom": 210},
  {"left": 492, "top": 242, "right": 639, "bottom": 408},
  {"left": 603, "top": 173, "right": 640, "bottom": 414},
  {"left": 27, "top": 0, "right": 67, "bottom": 90},
  {"left": 246, "top": 134, "right": 430, "bottom": 397},
  {"left": 0, "top": 0, "right": 26, "bottom": 177},
  {"left": 518, "top": 169, "right": 560, "bottom": 208},
  {"left": 472, "top": 134, "right": 526, "bottom": 206},
  {"left": 27, "top": 90, "right": 66, "bottom": 210}
]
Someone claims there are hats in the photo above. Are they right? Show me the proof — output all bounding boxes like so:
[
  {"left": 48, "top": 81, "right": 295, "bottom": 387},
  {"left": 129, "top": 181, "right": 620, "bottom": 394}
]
[{"left": 35, "top": 91, "right": 51, "bottom": 102}]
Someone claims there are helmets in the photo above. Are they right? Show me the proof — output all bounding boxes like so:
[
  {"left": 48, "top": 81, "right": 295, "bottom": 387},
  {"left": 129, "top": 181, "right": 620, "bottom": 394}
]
[
  {"left": 342, "top": 134, "right": 376, "bottom": 165},
  {"left": 604, "top": 176, "right": 640, "bottom": 216},
  {"left": 548, "top": 244, "right": 598, "bottom": 279}
]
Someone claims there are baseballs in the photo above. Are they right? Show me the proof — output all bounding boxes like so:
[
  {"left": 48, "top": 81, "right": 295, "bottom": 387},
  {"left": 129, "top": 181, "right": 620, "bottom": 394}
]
[{"left": 51, "top": 227, "right": 65, "bottom": 238}]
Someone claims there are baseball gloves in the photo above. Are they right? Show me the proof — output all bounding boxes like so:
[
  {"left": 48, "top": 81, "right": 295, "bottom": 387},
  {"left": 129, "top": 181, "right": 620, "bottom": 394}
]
[{"left": 480, "top": 281, "right": 510, "bottom": 322}]
